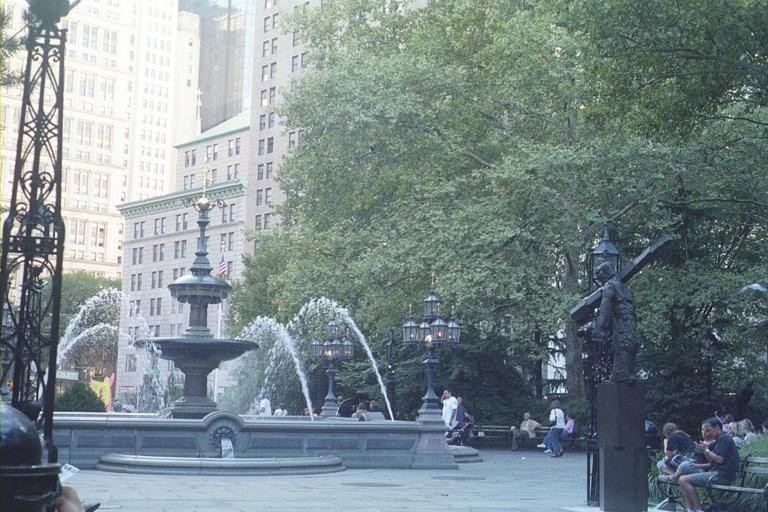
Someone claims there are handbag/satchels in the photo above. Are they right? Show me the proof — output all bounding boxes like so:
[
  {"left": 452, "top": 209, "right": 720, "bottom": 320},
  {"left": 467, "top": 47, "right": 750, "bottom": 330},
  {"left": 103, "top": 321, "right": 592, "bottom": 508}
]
[{"left": 547, "top": 409, "right": 556, "bottom": 427}]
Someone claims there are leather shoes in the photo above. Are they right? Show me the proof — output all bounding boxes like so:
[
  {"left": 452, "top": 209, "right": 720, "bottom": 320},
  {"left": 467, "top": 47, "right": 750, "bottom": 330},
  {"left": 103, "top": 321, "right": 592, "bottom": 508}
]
[{"left": 83, "top": 503, "right": 99, "bottom": 512}]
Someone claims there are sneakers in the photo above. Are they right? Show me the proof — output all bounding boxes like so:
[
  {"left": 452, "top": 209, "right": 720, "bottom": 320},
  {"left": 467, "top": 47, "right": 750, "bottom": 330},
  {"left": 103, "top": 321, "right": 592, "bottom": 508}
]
[
  {"left": 536, "top": 444, "right": 545, "bottom": 448},
  {"left": 658, "top": 474, "right": 676, "bottom": 484},
  {"left": 543, "top": 450, "right": 551, "bottom": 453}
]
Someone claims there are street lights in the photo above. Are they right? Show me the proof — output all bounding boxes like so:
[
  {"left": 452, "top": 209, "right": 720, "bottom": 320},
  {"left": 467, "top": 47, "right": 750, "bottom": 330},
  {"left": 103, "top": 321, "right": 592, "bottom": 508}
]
[
  {"left": 402, "top": 264, "right": 462, "bottom": 469},
  {"left": 311, "top": 313, "right": 354, "bottom": 417}
]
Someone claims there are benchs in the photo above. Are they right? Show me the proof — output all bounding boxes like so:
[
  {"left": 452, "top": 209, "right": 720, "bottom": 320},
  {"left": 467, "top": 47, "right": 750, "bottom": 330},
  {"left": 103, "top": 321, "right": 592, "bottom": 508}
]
[
  {"left": 655, "top": 456, "right": 768, "bottom": 512},
  {"left": 470, "top": 426, "right": 552, "bottom": 451}
]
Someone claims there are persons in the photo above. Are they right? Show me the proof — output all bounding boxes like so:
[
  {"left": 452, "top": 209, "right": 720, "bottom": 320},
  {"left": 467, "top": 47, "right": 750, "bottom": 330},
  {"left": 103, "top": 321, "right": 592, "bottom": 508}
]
[
  {"left": 456, "top": 394, "right": 473, "bottom": 446},
  {"left": 20, "top": 400, "right": 86, "bottom": 512},
  {"left": 657, "top": 411, "right": 768, "bottom": 512},
  {"left": 536, "top": 413, "right": 575, "bottom": 453},
  {"left": 510, "top": 412, "right": 541, "bottom": 450},
  {"left": 549, "top": 400, "right": 565, "bottom": 457},
  {"left": 595, "top": 262, "right": 641, "bottom": 382},
  {"left": 440, "top": 389, "right": 458, "bottom": 436},
  {"left": 257, "top": 394, "right": 379, "bottom": 418}
]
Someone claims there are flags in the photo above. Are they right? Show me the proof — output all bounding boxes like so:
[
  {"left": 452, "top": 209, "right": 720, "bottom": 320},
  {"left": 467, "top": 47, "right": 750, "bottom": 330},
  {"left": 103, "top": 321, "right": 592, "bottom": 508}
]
[{"left": 217, "top": 253, "right": 227, "bottom": 279}]
